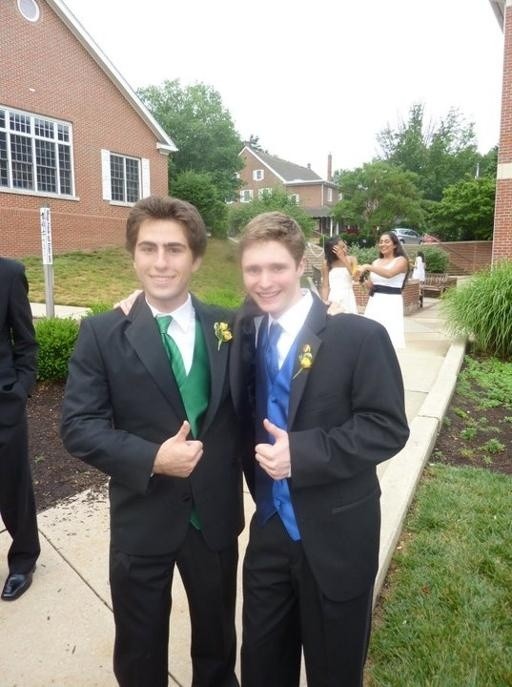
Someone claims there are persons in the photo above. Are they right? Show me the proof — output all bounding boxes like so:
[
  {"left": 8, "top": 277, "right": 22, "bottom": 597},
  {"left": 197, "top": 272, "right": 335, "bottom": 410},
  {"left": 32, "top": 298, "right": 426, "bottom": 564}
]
[
  {"left": 112, "top": 212, "right": 411, "bottom": 685},
  {"left": 321, "top": 236, "right": 359, "bottom": 316},
  {"left": 355, "top": 231, "right": 410, "bottom": 349},
  {"left": 58, "top": 196, "right": 352, "bottom": 687},
  {"left": 410, "top": 251, "right": 425, "bottom": 295},
  {"left": 0, "top": 257, "right": 41, "bottom": 600}
]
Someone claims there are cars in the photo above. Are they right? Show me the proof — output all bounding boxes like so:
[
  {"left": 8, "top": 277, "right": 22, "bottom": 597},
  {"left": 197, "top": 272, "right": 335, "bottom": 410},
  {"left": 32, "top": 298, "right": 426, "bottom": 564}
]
[{"left": 388, "top": 226, "right": 423, "bottom": 244}]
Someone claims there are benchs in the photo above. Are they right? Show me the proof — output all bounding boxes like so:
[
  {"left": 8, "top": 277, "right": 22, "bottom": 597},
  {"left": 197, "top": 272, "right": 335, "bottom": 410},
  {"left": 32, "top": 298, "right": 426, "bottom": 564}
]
[
  {"left": 447, "top": 275, "right": 457, "bottom": 287},
  {"left": 424, "top": 272, "right": 447, "bottom": 299}
]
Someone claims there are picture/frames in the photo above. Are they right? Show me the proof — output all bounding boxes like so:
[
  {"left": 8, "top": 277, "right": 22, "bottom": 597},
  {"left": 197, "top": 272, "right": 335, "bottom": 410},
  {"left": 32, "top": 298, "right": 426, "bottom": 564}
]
[{"left": 346, "top": 262, "right": 373, "bottom": 295}]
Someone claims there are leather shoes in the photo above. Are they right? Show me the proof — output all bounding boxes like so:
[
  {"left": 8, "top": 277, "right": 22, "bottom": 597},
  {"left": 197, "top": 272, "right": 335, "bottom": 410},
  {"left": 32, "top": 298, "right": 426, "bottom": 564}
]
[{"left": 1, "top": 563, "right": 35, "bottom": 600}]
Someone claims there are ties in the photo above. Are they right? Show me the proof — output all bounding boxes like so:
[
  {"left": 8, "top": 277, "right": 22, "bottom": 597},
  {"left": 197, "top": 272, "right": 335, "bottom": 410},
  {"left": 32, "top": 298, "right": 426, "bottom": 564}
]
[
  {"left": 154, "top": 316, "right": 185, "bottom": 386},
  {"left": 263, "top": 323, "right": 283, "bottom": 383}
]
